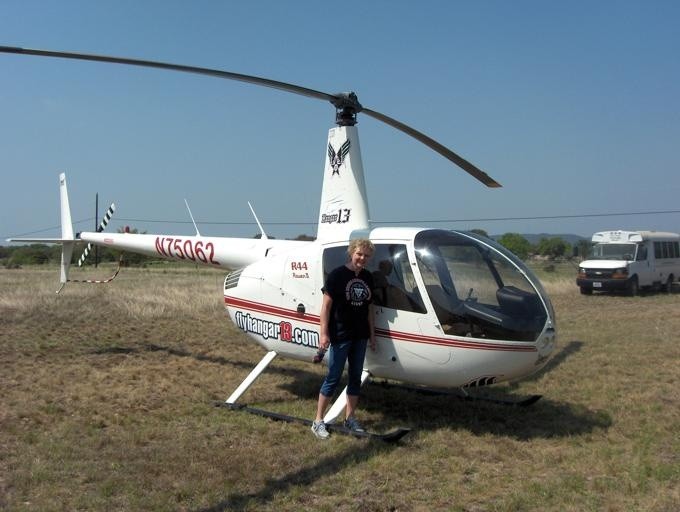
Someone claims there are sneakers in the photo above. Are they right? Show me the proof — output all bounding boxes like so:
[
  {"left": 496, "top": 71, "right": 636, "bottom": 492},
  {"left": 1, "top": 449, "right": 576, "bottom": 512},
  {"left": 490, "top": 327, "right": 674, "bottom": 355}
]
[
  {"left": 311, "top": 420, "right": 330, "bottom": 438},
  {"left": 344, "top": 418, "right": 365, "bottom": 432}
]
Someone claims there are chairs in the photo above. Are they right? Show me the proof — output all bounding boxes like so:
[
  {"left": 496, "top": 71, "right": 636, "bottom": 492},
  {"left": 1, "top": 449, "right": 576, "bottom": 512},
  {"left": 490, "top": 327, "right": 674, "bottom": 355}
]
[{"left": 413, "top": 285, "right": 460, "bottom": 322}]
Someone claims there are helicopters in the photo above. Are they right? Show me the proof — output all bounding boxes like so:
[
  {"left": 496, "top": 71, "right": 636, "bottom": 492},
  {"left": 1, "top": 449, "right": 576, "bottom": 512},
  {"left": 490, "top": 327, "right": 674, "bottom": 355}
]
[{"left": 0, "top": 45, "right": 556, "bottom": 442}]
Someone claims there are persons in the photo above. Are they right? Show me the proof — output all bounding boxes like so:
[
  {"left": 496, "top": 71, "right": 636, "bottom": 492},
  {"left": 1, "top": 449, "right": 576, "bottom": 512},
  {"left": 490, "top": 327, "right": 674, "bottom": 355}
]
[
  {"left": 310, "top": 238, "right": 378, "bottom": 440},
  {"left": 372, "top": 259, "right": 393, "bottom": 307}
]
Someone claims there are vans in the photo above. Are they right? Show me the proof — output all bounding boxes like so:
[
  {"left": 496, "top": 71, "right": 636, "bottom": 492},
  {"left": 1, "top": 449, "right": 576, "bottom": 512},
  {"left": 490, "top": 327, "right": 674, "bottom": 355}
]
[{"left": 576, "top": 231, "right": 680, "bottom": 296}]
